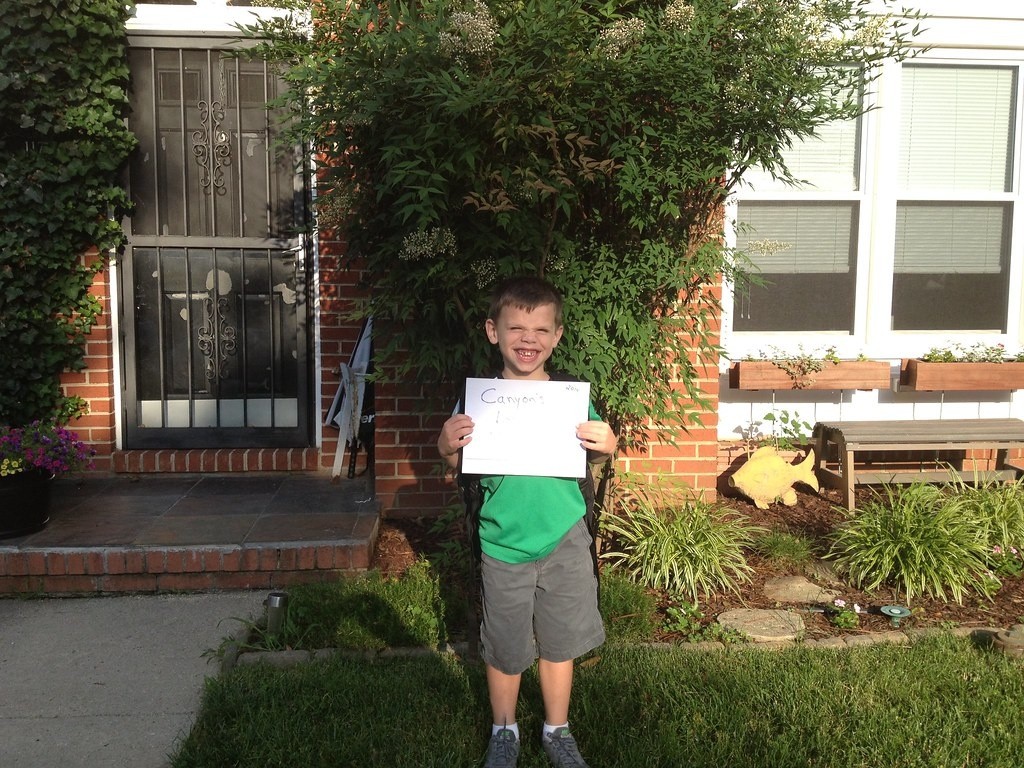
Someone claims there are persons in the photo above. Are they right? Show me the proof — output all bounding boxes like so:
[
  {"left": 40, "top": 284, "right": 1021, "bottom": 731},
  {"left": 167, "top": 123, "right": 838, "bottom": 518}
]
[{"left": 437, "top": 275, "right": 618, "bottom": 768}]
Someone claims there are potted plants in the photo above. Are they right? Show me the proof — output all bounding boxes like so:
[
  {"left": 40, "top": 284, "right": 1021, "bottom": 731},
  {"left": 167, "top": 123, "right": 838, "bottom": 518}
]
[{"left": 728, "top": 357, "right": 890, "bottom": 390}]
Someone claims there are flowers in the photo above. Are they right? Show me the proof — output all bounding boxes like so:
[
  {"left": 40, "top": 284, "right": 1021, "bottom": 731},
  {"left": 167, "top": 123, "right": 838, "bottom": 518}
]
[
  {"left": 0, "top": 415, "right": 101, "bottom": 476},
  {"left": 926, "top": 343, "right": 1024, "bottom": 363}
]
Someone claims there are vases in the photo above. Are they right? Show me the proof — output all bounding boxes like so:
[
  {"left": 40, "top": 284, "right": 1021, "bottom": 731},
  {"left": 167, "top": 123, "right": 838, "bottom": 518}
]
[
  {"left": 900, "top": 358, "right": 1023, "bottom": 391},
  {"left": 0, "top": 466, "right": 57, "bottom": 538}
]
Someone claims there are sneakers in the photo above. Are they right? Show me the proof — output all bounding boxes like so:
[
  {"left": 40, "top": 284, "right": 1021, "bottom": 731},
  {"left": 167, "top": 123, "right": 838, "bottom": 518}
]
[
  {"left": 484, "top": 716, "right": 520, "bottom": 768},
  {"left": 542, "top": 727, "right": 591, "bottom": 768}
]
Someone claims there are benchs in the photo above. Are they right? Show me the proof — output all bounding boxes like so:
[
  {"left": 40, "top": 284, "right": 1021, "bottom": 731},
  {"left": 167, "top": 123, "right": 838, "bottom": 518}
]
[{"left": 813, "top": 418, "right": 1023, "bottom": 519}]
